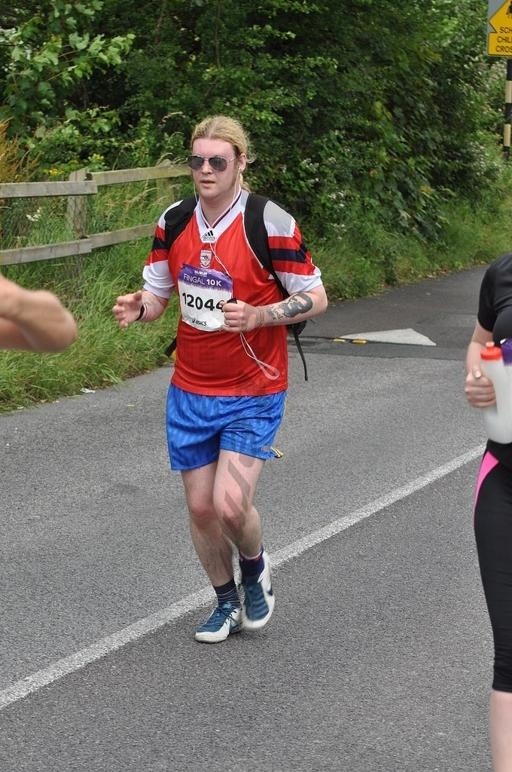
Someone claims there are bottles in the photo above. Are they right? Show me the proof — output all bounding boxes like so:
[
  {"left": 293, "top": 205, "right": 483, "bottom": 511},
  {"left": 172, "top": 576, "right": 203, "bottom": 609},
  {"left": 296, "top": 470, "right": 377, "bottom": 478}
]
[{"left": 478, "top": 339, "right": 512, "bottom": 446}]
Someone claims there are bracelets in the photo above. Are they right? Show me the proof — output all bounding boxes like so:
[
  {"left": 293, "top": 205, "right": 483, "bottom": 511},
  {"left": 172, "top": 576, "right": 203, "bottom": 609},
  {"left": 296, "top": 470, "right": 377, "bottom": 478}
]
[{"left": 135, "top": 303, "right": 146, "bottom": 321}]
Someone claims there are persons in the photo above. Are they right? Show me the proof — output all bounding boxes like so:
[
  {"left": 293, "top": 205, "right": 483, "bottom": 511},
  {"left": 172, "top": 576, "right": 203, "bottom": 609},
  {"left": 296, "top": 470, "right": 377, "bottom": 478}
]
[
  {"left": 276, "top": 292, "right": 314, "bottom": 319},
  {"left": 0, "top": 271, "right": 80, "bottom": 356},
  {"left": 461, "top": 250, "right": 512, "bottom": 771},
  {"left": 113, "top": 116, "right": 329, "bottom": 643}
]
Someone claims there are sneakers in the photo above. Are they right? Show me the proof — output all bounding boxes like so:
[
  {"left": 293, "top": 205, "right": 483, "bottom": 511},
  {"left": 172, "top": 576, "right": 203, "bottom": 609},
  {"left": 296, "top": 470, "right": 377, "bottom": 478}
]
[
  {"left": 239, "top": 550, "right": 275, "bottom": 628},
  {"left": 195, "top": 601, "right": 243, "bottom": 643}
]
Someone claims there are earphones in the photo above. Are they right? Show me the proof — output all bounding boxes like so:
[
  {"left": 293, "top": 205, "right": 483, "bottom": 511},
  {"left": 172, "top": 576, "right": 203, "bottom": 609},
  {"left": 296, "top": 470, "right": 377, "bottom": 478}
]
[{"left": 237, "top": 163, "right": 241, "bottom": 170}]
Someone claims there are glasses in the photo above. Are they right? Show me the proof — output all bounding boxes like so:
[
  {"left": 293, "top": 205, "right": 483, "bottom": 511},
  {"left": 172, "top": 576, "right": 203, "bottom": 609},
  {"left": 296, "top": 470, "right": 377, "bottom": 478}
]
[{"left": 187, "top": 156, "right": 237, "bottom": 171}]
